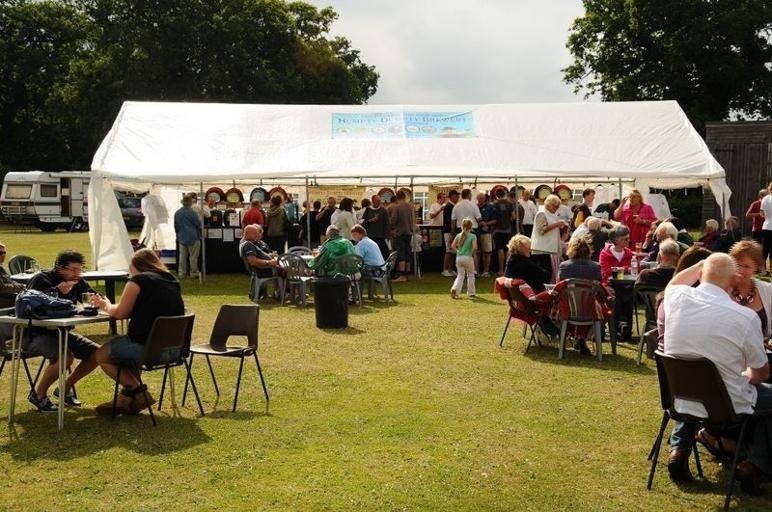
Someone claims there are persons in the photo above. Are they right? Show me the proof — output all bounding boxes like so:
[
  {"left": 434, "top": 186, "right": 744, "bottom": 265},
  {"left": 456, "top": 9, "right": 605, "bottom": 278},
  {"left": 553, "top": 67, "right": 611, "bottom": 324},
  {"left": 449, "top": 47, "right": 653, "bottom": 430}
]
[
  {"left": 307, "top": 224, "right": 361, "bottom": 304},
  {"left": 239, "top": 224, "right": 291, "bottom": 299},
  {"left": 173, "top": 195, "right": 203, "bottom": 280},
  {"left": 614, "top": 189, "right": 656, "bottom": 247},
  {"left": 241, "top": 194, "right": 389, "bottom": 259},
  {"left": 503, "top": 219, "right": 694, "bottom": 353},
  {"left": 389, "top": 190, "right": 412, "bottom": 283},
  {"left": 654, "top": 354, "right": 771, "bottom": 495},
  {"left": 572, "top": 188, "right": 620, "bottom": 259},
  {"left": 188, "top": 192, "right": 211, "bottom": 233},
  {"left": 90, "top": 247, "right": 186, "bottom": 417},
  {"left": 428, "top": 187, "right": 536, "bottom": 277},
  {"left": 697, "top": 186, "right": 770, "bottom": 253},
  {"left": 531, "top": 194, "right": 565, "bottom": 280},
  {"left": 450, "top": 219, "right": 477, "bottom": 297},
  {"left": 351, "top": 224, "right": 386, "bottom": 306},
  {"left": 12, "top": 249, "right": 108, "bottom": 414},
  {"left": 727, "top": 239, "right": 769, "bottom": 350},
  {"left": 0, "top": 242, "right": 28, "bottom": 306}
]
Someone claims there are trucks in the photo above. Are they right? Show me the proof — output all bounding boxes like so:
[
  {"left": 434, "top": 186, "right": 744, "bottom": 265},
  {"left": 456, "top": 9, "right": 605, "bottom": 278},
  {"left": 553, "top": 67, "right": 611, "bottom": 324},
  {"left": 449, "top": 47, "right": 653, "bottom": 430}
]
[{"left": 0, "top": 171, "right": 103, "bottom": 234}]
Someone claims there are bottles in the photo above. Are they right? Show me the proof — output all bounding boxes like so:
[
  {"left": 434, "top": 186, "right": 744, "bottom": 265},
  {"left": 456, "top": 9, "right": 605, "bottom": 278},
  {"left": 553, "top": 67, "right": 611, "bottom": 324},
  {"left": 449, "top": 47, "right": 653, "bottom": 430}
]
[{"left": 630, "top": 255, "right": 638, "bottom": 276}]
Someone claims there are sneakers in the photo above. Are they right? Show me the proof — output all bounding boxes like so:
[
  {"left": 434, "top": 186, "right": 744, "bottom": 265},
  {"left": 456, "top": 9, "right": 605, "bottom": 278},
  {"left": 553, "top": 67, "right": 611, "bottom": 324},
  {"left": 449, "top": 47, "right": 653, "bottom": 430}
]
[
  {"left": 28, "top": 393, "right": 57, "bottom": 413},
  {"left": 441, "top": 270, "right": 504, "bottom": 277},
  {"left": 52, "top": 387, "right": 81, "bottom": 408},
  {"left": 573, "top": 339, "right": 590, "bottom": 355}
]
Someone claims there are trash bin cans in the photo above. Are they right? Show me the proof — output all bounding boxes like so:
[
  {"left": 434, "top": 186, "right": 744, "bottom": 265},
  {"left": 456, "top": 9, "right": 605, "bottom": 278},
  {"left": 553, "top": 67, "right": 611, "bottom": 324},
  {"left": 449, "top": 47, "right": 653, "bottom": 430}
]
[{"left": 311, "top": 277, "right": 351, "bottom": 328}]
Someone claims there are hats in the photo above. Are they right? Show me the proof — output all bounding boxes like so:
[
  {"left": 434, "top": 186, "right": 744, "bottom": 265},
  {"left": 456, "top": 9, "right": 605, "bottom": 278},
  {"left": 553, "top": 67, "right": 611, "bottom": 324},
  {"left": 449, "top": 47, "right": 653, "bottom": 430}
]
[
  {"left": 181, "top": 195, "right": 196, "bottom": 204},
  {"left": 449, "top": 190, "right": 460, "bottom": 196}
]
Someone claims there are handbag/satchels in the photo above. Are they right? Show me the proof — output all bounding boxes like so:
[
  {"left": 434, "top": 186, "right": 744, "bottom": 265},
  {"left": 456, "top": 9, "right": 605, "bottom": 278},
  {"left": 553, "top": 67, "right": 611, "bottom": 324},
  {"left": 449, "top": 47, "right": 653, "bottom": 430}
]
[{"left": 480, "top": 233, "right": 492, "bottom": 252}]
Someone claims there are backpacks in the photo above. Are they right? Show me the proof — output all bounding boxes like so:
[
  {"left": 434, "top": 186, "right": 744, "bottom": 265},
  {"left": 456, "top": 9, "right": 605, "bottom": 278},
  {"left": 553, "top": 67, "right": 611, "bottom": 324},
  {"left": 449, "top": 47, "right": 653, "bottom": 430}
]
[{"left": 15, "top": 289, "right": 77, "bottom": 321}]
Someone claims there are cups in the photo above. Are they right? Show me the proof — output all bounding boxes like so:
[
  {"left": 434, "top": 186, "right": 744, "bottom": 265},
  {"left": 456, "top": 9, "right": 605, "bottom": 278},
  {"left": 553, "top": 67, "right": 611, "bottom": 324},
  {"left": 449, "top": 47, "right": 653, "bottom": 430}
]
[
  {"left": 635, "top": 243, "right": 642, "bottom": 252},
  {"left": 79, "top": 293, "right": 106, "bottom": 316},
  {"left": 610, "top": 267, "right": 624, "bottom": 281},
  {"left": 312, "top": 246, "right": 323, "bottom": 258}
]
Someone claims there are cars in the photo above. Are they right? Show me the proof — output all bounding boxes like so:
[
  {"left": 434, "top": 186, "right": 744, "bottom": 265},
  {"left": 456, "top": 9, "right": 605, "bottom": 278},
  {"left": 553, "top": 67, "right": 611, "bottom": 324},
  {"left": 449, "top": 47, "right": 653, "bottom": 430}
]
[{"left": 117, "top": 195, "right": 145, "bottom": 225}]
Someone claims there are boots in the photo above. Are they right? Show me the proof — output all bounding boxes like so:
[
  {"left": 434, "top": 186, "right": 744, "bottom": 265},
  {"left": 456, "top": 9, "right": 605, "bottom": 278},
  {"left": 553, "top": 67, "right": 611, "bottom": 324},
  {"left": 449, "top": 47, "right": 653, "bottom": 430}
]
[{"left": 96, "top": 384, "right": 155, "bottom": 416}]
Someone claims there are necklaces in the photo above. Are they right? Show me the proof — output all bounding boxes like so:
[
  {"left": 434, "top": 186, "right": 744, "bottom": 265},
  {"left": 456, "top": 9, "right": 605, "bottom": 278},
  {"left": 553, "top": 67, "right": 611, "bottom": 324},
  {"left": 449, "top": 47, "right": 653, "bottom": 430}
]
[{"left": 729, "top": 284, "right": 756, "bottom": 305}]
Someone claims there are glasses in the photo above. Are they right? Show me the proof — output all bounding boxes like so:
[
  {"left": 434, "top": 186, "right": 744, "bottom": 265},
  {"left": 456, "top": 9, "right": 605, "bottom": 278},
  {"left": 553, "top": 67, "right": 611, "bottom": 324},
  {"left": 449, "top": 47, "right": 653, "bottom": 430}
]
[{"left": 0, "top": 251, "right": 6, "bottom": 255}]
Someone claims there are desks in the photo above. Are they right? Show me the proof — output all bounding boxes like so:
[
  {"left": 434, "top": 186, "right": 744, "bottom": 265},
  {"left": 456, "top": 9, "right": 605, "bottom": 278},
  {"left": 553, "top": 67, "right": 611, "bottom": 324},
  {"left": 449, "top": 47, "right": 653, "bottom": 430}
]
[
  {"left": 10, "top": 271, "right": 130, "bottom": 337},
  {"left": 606, "top": 277, "right": 635, "bottom": 341},
  {"left": 1, "top": 311, "right": 114, "bottom": 434}
]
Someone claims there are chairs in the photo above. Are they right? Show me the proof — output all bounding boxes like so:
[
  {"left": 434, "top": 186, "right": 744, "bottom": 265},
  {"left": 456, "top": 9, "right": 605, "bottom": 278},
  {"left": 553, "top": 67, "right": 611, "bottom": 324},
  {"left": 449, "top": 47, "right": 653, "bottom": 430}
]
[
  {"left": 2, "top": 307, "right": 77, "bottom": 413},
  {"left": 634, "top": 282, "right": 665, "bottom": 366},
  {"left": 249, "top": 247, "right": 399, "bottom": 307},
  {"left": 8, "top": 255, "right": 36, "bottom": 275},
  {"left": 181, "top": 305, "right": 267, "bottom": 413},
  {"left": 550, "top": 279, "right": 608, "bottom": 362},
  {"left": 497, "top": 278, "right": 548, "bottom": 353},
  {"left": 646, "top": 350, "right": 771, "bottom": 506},
  {"left": 112, "top": 313, "right": 203, "bottom": 426}
]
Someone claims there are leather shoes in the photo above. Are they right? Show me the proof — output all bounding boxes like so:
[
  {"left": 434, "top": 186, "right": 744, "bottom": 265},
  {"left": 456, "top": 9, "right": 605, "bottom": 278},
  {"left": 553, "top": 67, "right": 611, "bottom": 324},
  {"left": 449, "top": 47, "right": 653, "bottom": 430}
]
[
  {"left": 668, "top": 449, "right": 695, "bottom": 482},
  {"left": 734, "top": 462, "right": 762, "bottom": 496}
]
